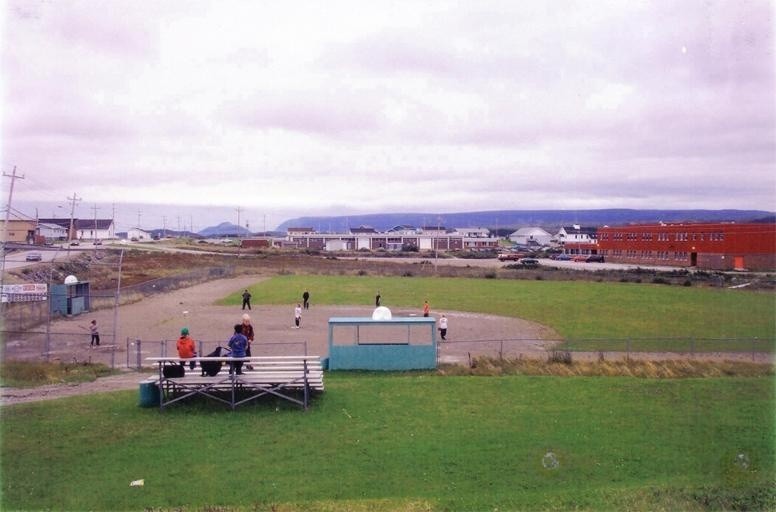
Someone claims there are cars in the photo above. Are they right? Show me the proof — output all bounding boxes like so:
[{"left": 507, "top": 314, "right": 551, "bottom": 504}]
[
  {"left": 69, "top": 239, "right": 78, "bottom": 246},
  {"left": 23, "top": 252, "right": 42, "bottom": 261},
  {"left": 92, "top": 238, "right": 102, "bottom": 245},
  {"left": 499, "top": 252, "right": 605, "bottom": 266}
]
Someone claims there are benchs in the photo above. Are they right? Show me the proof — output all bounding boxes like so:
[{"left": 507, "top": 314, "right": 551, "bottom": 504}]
[{"left": 144, "top": 353, "right": 326, "bottom": 413}]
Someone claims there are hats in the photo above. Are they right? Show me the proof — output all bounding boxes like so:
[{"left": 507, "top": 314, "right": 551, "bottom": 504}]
[{"left": 181, "top": 328, "right": 188, "bottom": 335}]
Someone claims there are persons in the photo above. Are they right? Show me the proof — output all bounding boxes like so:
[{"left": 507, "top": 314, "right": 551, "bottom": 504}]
[
  {"left": 294, "top": 303, "right": 301, "bottom": 328},
  {"left": 176, "top": 326, "right": 197, "bottom": 372},
  {"left": 423, "top": 300, "right": 431, "bottom": 317},
  {"left": 240, "top": 289, "right": 252, "bottom": 311},
  {"left": 375, "top": 291, "right": 381, "bottom": 308},
  {"left": 240, "top": 314, "right": 255, "bottom": 370},
  {"left": 302, "top": 288, "right": 309, "bottom": 313},
  {"left": 228, "top": 324, "right": 248, "bottom": 376},
  {"left": 88, "top": 320, "right": 100, "bottom": 346},
  {"left": 439, "top": 314, "right": 448, "bottom": 340}
]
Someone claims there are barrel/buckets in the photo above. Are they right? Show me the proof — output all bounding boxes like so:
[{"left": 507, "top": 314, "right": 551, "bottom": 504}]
[{"left": 140, "top": 381, "right": 161, "bottom": 406}]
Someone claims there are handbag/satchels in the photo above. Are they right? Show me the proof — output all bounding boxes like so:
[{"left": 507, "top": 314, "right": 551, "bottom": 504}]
[
  {"left": 163, "top": 364, "right": 184, "bottom": 378},
  {"left": 200, "top": 347, "right": 222, "bottom": 376}
]
